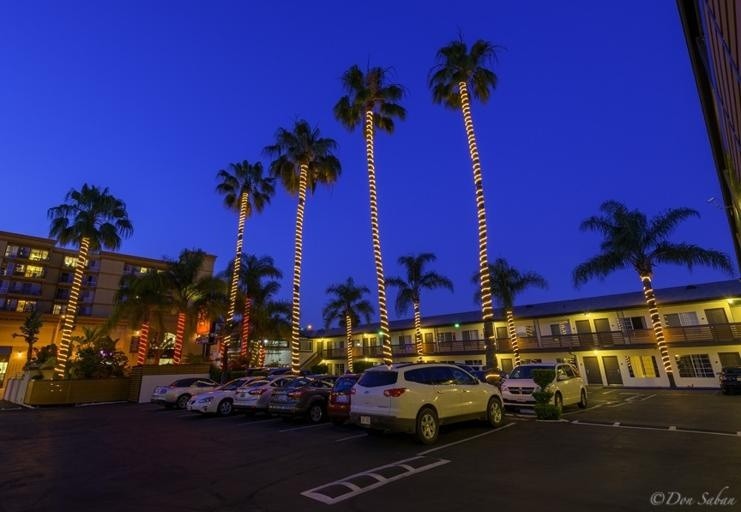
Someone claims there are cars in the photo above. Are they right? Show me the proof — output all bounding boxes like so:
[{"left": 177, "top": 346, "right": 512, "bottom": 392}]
[{"left": 716, "top": 366, "right": 741, "bottom": 394}]
